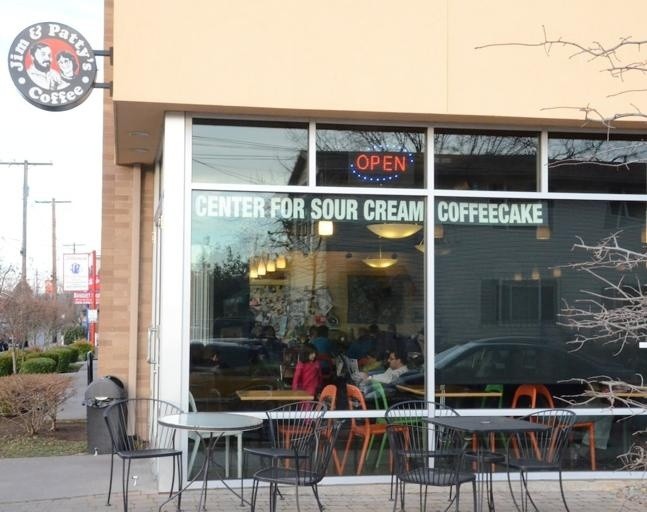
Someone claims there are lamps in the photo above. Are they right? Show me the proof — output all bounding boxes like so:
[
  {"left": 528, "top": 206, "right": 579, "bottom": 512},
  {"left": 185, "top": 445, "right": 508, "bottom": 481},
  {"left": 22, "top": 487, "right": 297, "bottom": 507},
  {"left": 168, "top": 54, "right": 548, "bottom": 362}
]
[
  {"left": 249, "top": 265, "right": 258, "bottom": 278},
  {"left": 536, "top": 226, "right": 550, "bottom": 241},
  {"left": 266, "top": 257, "right": 277, "bottom": 272},
  {"left": 257, "top": 261, "right": 267, "bottom": 276},
  {"left": 363, "top": 242, "right": 399, "bottom": 268},
  {"left": 553, "top": 266, "right": 561, "bottom": 278},
  {"left": 366, "top": 224, "right": 424, "bottom": 239},
  {"left": 514, "top": 273, "right": 523, "bottom": 282},
  {"left": 276, "top": 254, "right": 286, "bottom": 269},
  {"left": 532, "top": 267, "right": 541, "bottom": 280}
]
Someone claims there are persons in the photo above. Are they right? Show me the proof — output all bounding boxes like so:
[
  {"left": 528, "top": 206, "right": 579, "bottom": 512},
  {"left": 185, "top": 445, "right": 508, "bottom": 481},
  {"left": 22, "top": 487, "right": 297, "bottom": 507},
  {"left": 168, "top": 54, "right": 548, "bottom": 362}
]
[
  {"left": 53, "top": 49, "right": 80, "bottom": 91},
  {"left": 26, "top": 41, "right": 61, "bottom": 91},
  {"left": 201, "top": 323, "right": 425, "bottom": 400},
  {"left": 0, "top": 330, "right": 29, "bottom": 352}
]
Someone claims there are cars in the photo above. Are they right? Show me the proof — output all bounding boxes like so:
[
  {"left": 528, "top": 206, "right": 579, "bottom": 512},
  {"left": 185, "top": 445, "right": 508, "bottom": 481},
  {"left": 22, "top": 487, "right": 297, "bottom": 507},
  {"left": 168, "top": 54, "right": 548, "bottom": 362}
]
[
  {"left": 358, "top": 333, "right": 647, "bottom": 470},
  {"left": 189, "top": 333, "right": 330, "bottom": 417}
]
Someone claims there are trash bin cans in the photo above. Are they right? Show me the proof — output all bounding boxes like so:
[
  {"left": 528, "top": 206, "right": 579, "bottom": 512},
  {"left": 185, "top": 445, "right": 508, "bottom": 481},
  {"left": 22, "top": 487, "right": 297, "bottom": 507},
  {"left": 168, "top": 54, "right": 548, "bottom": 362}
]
[{"left": 81, "top": 375, "right": 128, "bottom": 454}]
